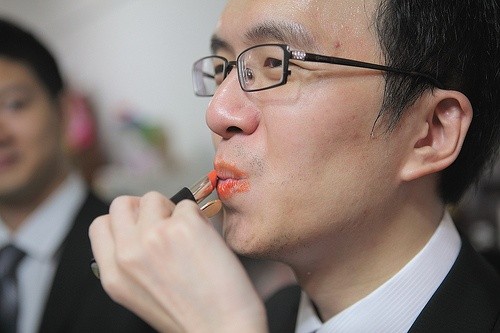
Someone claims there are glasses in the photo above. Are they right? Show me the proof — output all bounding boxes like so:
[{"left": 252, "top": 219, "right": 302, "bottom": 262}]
[{"left": 192, "top": 41, "right": 447, "bottom": 97}]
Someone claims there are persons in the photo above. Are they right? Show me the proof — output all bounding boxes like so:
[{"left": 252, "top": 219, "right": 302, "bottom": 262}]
[
  {"left": 87, "top": 0, "right": 500, "bottom": 333},
  {"left": 0, "top": 17, "right": 156, "bottom": 333}
]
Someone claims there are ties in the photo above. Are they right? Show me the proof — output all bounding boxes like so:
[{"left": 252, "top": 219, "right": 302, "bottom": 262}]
[{"left": 0, "top": 244, "right": 28, "bottom": 333}]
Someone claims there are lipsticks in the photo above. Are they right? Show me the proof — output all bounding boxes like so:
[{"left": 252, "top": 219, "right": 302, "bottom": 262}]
[{"left": 168, "top": 169, "right": 225, "bottom": 220}]
